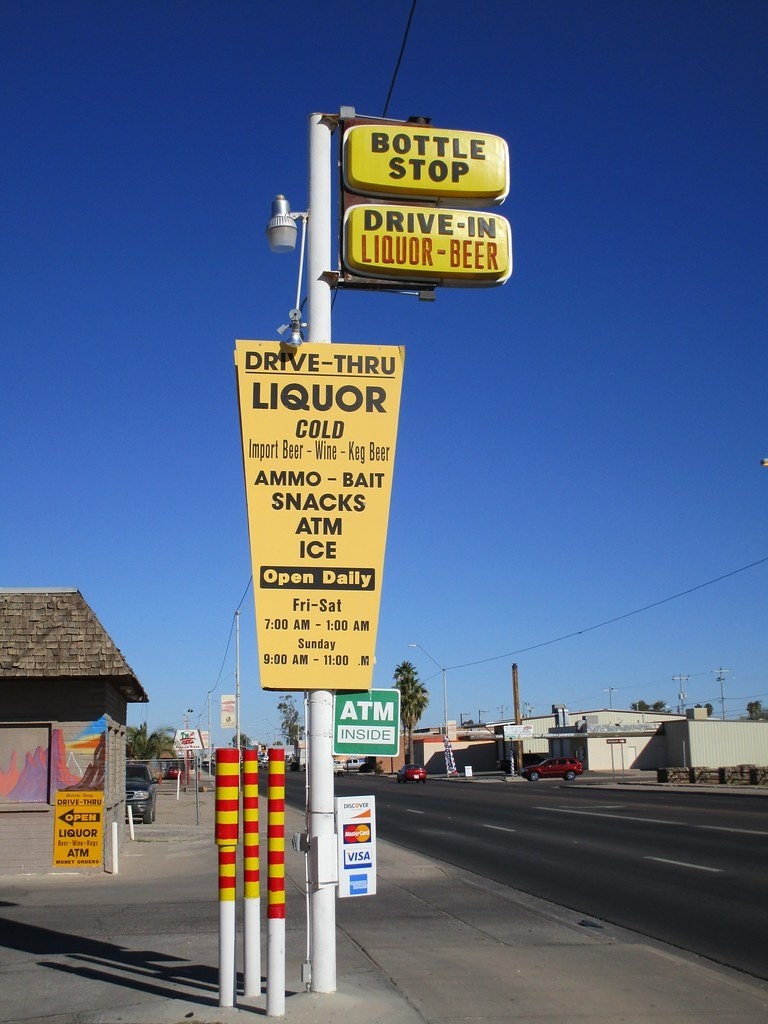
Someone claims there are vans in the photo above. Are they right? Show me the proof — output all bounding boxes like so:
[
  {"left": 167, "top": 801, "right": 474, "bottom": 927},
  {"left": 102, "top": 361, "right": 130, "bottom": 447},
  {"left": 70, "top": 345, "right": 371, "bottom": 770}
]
[{"left": 342, "top": 758, "right": 366, "bottom": 769}]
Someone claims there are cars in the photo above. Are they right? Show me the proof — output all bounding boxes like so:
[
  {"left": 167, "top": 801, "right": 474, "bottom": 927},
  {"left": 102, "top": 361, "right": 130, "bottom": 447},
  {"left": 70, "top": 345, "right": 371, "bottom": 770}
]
[
  {"left": 333, "top": 760, "right": 345, "bottom": 777},
  {"left": 203, "top": 761, "right": 209, "bottom": 767},
  {"left": 396, "top": 764, "right": 427, "bottom": 784},
  {"left": 211, "top": 759, "right": 268, "bottom": 769},
  {"left": 163, "top": 766, "right": 183, "bottom": 779},
  {"left": 496, "top": 752, "right": 546, "bottom": 774}
]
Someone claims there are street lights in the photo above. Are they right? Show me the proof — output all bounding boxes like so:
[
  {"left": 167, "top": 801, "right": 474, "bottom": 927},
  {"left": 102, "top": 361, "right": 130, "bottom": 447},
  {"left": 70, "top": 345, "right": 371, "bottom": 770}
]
[
  {"left": 407, "top": 644, "right": 448, "bottom": 737},
  {"left": 509, "top": 737, "right": 513, "bottom": 755}
]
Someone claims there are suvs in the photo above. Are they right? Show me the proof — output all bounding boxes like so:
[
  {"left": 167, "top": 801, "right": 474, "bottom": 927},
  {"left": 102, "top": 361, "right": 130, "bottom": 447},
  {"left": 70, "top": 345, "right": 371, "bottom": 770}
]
[
  {"left": 125, "top": 764, "right": 159, "bottom": 825},
  {"left": 522, "top": 757, "right": 584, "bottom": 782}
]
